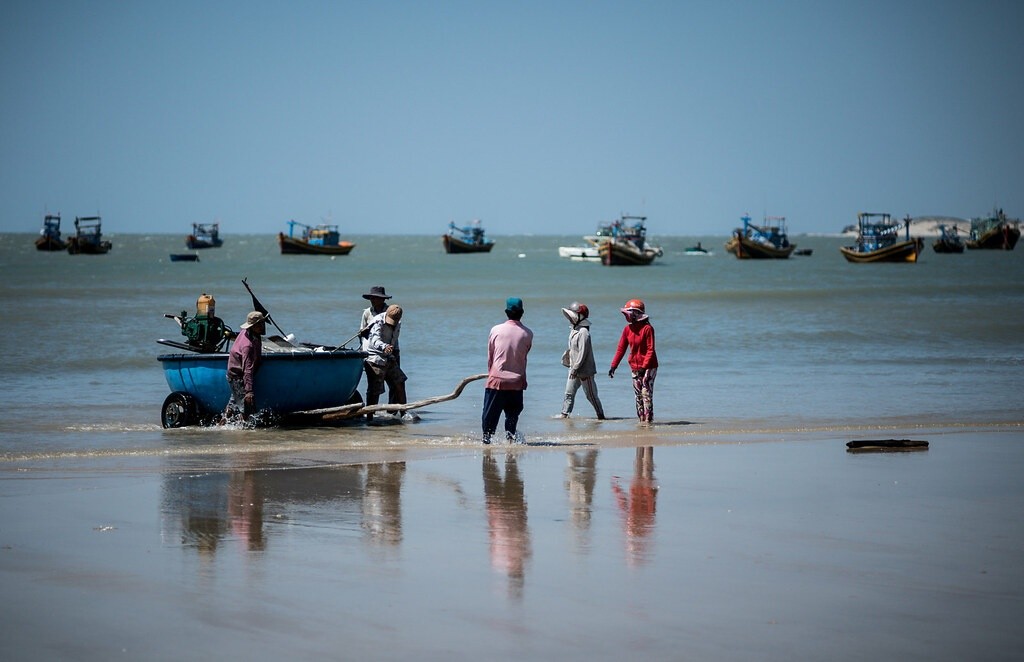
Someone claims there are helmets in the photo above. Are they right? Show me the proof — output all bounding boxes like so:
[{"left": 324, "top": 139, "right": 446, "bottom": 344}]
[
  {"left": 569, "top": 303, "right": 588, "bottom": 318},
  {"left": 625, "top": 299, "right": 644, "bottom": 313}
]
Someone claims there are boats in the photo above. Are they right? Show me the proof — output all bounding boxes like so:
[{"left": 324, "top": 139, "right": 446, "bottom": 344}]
[
  {"left": 724, "top": 213, "right": 797, "bottom": 260},
  {"left": 684, "top": 246, "right": 707, "bottom": 253},
  {"left": 558, "top": 215, "right": 663, "bottom": 266},
  {"left": 839, "top": 211, "right": 925, "bottom": 264},
  {"left": 34, "top": 214, "right": 113, "bottom": 255},
  {"left": 156, "top": 342, "right": 370, "bottom": 416},
  {"left": 279, "top": 220, "right": 354, "bottom": 256},
  {"left": 931, "top": 223, "right": 965, "bottom": 254},
  {"left": 792, "top": 249, "right": 813, "bottom": 257},
  {"left": 964, "top": 210, "right": 1021, "bottom": 250},
  {"left": 440, "top": 220, "right": 496, "bottom": 253},
  {"left": 169, "top": 253, "right": 201, "bottom": 262},
  {"left": 185, "top": 222, "right": 223, "bottom": 250}
]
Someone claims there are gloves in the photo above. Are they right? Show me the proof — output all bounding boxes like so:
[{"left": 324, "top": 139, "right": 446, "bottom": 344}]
[
  {"left": 638, "top": 367, "right": 646, "bottom": 377},
  {"left": 608, "top": 367, "right": 616, "bottom": 378}
]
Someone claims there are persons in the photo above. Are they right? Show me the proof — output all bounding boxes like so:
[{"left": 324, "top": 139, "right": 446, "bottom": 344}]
[
  {"left": 219, "top": 311, "right": 266, "bottom": 425},
  {"left": 359, "top": 285, "right": 408, "bottom": 418},
  {"left": 608, "top": 300, "right": 658, "bottom": 421},
  {"left": 553, "top": 303, "right": 605, "bottom": 420},
  {"left": 482, "top": 296, "right": 532, "bottom": 442}
]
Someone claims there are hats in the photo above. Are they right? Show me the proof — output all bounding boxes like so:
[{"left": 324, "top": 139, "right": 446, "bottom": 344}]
[
  {"left": 240, "top": 312, "right": 270, "bottom": 329},
  {"left": 386, "top": 305, "right": 403, "bottom": 326},
  {"left": 561, "top": 308, "right": 592, "bottom": 327},
  {"left": 362, "top": 286, "right": 393, "bottom": 300},
  {"left": 506, "top": 296, "right": 523, "bottom": 311},
  {"left": 620, "top": 308, "right": 649, "bottom": 322}
]
